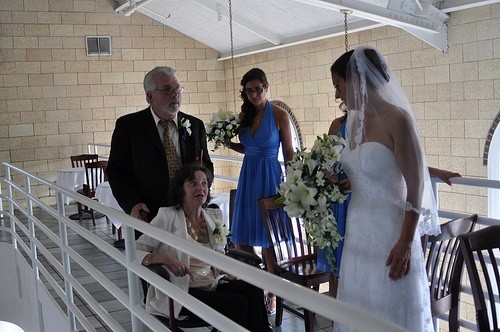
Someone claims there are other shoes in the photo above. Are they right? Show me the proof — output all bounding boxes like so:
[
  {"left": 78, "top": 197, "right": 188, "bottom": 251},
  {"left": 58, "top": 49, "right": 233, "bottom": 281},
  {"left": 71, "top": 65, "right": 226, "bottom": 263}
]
[{"left": 267, "top": 294, "right": 276, "bottom": 315}]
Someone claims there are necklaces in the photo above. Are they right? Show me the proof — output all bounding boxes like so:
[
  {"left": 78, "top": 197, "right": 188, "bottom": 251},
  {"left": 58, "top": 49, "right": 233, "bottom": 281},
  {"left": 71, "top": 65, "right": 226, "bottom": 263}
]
[
  {"left": 186, "top": 216, "right": 207, "bottom": 242},
  {"left": 362, "top": 97, "right": 383, "bottom": 124},
  {"left": 253, "top": 115, "right": 262, "bottom": 127}
]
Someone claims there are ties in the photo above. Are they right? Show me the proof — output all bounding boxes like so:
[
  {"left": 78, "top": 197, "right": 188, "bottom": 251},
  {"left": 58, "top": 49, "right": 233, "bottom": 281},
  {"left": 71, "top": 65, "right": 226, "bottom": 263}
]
[{"left": 160, "top": 120, "right": 182, "bottom": 181}]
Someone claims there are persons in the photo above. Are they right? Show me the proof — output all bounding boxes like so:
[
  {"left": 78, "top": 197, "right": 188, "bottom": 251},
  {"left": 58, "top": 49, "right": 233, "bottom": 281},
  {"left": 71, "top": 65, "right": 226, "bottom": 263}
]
[
  {"left": 220, "top": 68, "right": 294, "bottom": 316},
  {"left": 315, "top": 103, "right": 462, "bottom": 298},
  {"left": 331, "top": 45, "right": 436, "bottom": 332},
  {"left": 136, "top": 164, "right": 272, "bottom": 332},
  {"left": 105, "top": 66, "right": 214, "bottom": 304}
]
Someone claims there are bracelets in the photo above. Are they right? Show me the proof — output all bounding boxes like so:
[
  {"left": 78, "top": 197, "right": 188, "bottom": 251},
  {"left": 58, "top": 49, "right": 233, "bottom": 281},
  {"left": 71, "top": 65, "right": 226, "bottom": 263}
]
[{"left": 398, "top": 240, "right": 411, "bottom": 249}]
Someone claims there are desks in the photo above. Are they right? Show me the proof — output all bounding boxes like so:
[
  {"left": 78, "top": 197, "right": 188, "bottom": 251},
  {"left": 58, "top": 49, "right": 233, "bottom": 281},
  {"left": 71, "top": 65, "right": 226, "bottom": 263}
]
[
  {"left": 96, "top": 181, "right": 125, "bottom": 250},
  {"left": 57, "top": 167, "right": 106, "bottom": 220},
  {"left": 208, "top": 193, "right": 229, "bottom": 230}
]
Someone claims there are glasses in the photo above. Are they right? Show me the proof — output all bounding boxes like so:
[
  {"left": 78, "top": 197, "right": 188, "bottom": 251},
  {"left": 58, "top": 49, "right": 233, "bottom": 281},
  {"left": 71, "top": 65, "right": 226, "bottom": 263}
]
[
  {"left": 244, "top": 84, "right": 267, "bottom": 95},
  {"left": 153, "top": 86, "right": 184, "bottom": 96}
]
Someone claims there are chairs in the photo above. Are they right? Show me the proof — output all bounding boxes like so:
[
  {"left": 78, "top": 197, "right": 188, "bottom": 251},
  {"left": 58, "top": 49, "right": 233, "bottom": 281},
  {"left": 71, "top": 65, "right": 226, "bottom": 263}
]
[
  {"left": 420, "top": 214, "right": 500, "bottom": 332},
  {"left": 70, "top": 153, "right": 123, "bottom": 240},
  {"left": 138, "top": 189, "right": 339, "bottom": 332}
]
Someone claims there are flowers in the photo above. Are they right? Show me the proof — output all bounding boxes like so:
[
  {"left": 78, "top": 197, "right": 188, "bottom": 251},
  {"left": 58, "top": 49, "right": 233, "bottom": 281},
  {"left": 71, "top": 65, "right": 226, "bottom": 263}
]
[
  {"left": 205, "top": 108, "right": 241, "bottom": 152},
  {"left": 274, "top": 132, "right": 349, "bottom": 284},
  {"left": 214, "top": 218, "right": 234, "bottom": 243},
  {"left": 180, "top": 118, "right": 192, "bottom": 139}
]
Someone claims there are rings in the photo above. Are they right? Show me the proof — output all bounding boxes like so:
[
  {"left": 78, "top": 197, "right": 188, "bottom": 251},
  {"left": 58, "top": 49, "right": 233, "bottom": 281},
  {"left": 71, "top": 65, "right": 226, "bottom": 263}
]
[
  {"left": 403, "top": 265, "right": 407, "bottom": 267},
  {"left": 182, "top": 271, "right": 186, "bottom": 275}
]
